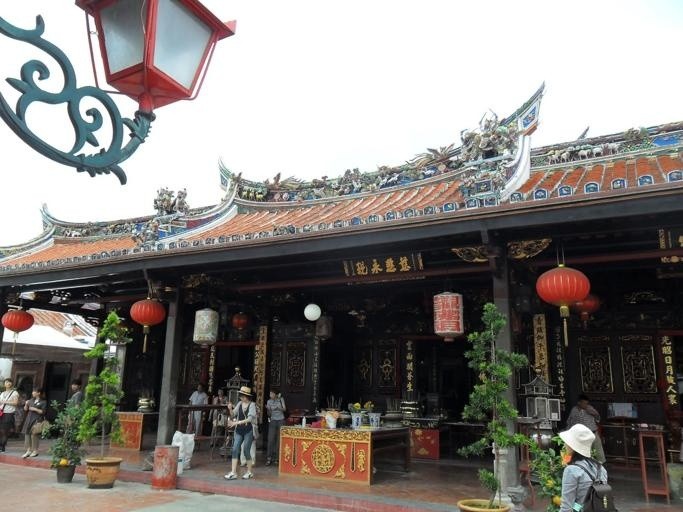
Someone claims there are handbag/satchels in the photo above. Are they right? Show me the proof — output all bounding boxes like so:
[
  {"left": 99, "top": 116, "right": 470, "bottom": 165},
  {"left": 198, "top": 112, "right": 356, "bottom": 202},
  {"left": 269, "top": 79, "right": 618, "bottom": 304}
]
[
  {"left": 0, "top": 408, "right": 3, "bottom": 417},
  {"left": 31, "top": 423, "right": 42, "bottom": 435}
]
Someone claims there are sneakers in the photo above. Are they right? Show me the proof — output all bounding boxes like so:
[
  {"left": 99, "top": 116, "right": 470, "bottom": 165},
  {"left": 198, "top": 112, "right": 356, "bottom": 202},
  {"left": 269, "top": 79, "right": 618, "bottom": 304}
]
[
  {"left": 224, "top": 472, "right": 237, "bottom": 480},
  {"left": 242, "top": 472, "right": 254, "bottom": 480},
  {"left": 22, "top": 450, "right": 39, "bottom": 458},
  {"left": 239, "top": 458, "right": 279, "bottom": 468}
]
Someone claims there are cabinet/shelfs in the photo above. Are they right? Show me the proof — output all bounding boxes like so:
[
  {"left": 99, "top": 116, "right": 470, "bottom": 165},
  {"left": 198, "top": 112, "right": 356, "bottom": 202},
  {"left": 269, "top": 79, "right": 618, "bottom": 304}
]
[
  {"left": 635, "top": 428, "right": 671, "bottom": 503},
  {"left": 175, "top": 403, "right": 230, "bottom": 458},
  {"left": 600, "top": 425, "right": 659, "bottom": 460}
]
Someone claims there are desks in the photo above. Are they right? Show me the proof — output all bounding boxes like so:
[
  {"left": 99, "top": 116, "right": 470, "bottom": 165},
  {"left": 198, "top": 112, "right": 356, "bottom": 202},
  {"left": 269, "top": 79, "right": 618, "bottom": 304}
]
[
  {"left": 277, "top": 424, "right": 410, "bottom": 488},
  {"left": 107, "top": 410, "right": 159, "bottom": 452}
]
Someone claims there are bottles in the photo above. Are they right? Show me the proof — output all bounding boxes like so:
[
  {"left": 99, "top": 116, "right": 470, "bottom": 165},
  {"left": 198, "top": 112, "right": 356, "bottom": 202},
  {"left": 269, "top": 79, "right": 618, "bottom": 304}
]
[{"left": 301, "top": 416, "right": 306, "bottom": 427}]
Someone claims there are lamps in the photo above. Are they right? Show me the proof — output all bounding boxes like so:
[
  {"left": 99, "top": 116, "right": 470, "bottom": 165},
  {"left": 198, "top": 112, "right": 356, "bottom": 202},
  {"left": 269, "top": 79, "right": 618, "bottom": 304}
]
[{"left": 303, "top": 303, "right": 322, "bottom": 322}]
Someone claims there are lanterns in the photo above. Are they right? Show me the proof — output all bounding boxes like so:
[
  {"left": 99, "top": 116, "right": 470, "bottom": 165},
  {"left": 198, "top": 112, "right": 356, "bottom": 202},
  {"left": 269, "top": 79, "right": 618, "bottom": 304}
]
[
  {"left": 1, "top": 307, "right": 34, "bottom": 354},
  {"left": 535, "top": 245, "right": 591, "bottom": 347},
  {"left": 129, "top": 291, "right": 166, "bottom": 354},
  {"left": 573, "top": 294, "right": 603, "bottom": 334},
  {"left": 231, "top": 310, "right": 249, "bottom": 343},
  {"left": 432, "top": 290, "right": 464, "bottom": 344},
  {"left": 314, "top": 310, "right": 336, "bottom": 343},
  {"left": 193, "top": 307, "right": 221, "bottom": 352}
]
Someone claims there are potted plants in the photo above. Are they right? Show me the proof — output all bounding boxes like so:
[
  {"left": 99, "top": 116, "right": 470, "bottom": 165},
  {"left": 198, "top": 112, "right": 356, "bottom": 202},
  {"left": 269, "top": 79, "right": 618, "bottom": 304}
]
[
  {"left": 74, "top": 311, "right": 133, "bottom": 491},
  {"left": 454, "top": 302, "right": 536, "bottom": 512},
  {"left": 39, "top": 398, "right": 86, "bottom": 485}
]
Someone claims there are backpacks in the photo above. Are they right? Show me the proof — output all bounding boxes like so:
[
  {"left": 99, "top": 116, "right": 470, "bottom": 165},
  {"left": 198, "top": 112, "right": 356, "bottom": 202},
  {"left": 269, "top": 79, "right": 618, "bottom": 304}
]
[{"left": 568, "top": 463, "right": 616, "bottom": 512}]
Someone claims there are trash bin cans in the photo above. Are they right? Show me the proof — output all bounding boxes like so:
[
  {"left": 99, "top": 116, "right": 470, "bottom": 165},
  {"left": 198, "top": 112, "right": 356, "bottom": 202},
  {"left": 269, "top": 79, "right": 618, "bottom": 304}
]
[{"left": 150, "top": 444, "right": 180, "bottom": 492}]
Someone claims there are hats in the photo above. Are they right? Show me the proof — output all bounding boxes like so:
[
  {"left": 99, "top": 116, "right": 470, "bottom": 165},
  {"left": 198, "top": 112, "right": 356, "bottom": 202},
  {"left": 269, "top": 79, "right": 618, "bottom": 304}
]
[
  {"left": 559, "top": 423, "right": 596, "bottom": 459},
  {"left": 237, "top": 386, "right": 253, "bottom": 396}
]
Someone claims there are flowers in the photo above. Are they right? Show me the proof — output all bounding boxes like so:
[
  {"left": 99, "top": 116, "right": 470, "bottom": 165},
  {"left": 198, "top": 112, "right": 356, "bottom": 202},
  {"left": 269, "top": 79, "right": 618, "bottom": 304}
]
[
  {"left": 347, "top": 402, "right": 362, "bottom": 413},
  {"left": 363, "top": 400, "right": 374, "bottom": 413}
]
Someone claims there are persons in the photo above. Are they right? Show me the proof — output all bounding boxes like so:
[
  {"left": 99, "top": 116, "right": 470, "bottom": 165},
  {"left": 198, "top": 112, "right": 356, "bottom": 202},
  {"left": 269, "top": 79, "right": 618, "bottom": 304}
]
[
  {"left": 565, "top": 395, "right": 606, "bottom": 466},
  {"left": 187, "top": 382, "right": 208, "bottom": 435},
  {"left": 208, "top": 387, "right": 228, "bottom": 448},
  {"left": 265, "top": 388, "right": 287, "bottom": 466},
  {"left": 69, "top": 379, "right": 83, "bottom": 409},
  {"left": 21, "top": 387, "right": 48, "bottom": 458},
  {"left": 0, "top": 378, "right": 19, "bottom": 452},
  {"left": 224, "top": 386, "right": 259, "bottom": 480},
  {"left": 554, "top": 422, "right": 616, "bottom": 512},
  {"left": 239, "top": 393, "right": 262, "bottom": 468}
]
